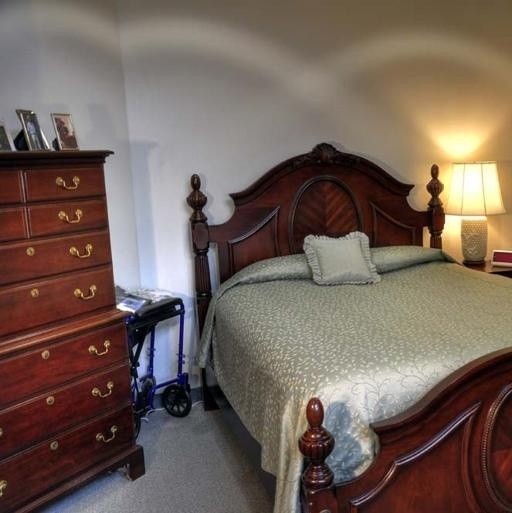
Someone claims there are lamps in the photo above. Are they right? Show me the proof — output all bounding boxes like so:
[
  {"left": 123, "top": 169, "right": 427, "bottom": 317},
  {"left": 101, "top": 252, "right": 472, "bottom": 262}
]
[{"left": 445, "top": 160, "right": 504, "bottom": 263}]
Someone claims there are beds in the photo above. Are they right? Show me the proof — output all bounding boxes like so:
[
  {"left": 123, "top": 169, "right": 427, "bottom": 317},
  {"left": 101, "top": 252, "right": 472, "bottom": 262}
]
[{"left": 188, "top": 144, "right": 512, "bottom": 513}]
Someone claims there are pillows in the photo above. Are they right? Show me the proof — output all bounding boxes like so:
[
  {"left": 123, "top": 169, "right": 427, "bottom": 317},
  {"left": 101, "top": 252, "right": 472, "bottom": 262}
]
[{"left": 301, "top": 230, "right": 381, "bottom": 287}]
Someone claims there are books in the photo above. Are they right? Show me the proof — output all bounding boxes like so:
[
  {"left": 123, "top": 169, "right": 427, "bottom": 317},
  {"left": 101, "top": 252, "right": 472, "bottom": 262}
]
[{"left": 115, "top": 292, "right": 147, "bottom": 312}]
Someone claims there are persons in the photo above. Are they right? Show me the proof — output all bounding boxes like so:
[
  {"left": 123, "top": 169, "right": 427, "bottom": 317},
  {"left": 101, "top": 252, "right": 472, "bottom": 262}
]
[
  {"left": 60, "top": 120, "right": 69, "bottom": 135},
  {"left": 27, "top": 114, "right": 42, "bottom": 150}
]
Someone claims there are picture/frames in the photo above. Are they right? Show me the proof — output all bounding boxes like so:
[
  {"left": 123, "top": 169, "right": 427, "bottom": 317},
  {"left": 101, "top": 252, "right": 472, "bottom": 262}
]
[{"left": 0, "top": 109, "right": 79, "bottom": 151}]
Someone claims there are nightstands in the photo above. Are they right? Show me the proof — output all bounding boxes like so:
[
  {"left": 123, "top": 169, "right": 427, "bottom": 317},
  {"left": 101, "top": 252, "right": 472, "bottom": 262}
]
[{"left": 458, "top": 261, "right": 512, "bottom": 280}]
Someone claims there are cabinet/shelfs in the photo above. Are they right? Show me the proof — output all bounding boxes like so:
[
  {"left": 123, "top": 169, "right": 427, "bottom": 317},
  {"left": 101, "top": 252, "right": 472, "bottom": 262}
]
[{"left": 0, "top": 150, "right": 148, "bottom": 513}]
